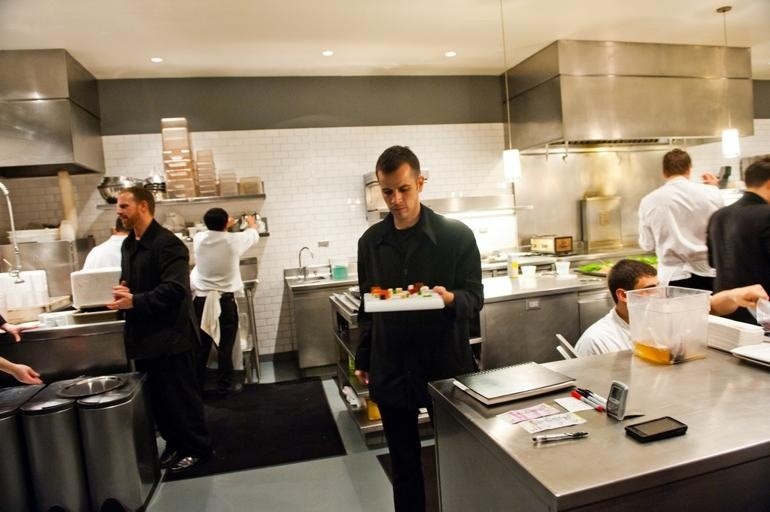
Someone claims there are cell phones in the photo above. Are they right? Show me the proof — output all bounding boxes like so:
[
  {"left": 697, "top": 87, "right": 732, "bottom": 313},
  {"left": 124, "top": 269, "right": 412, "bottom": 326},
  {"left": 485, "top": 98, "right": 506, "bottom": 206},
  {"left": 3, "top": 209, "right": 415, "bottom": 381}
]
[{"left": 624, "top": 415, "right": 688, "bottom": 443}]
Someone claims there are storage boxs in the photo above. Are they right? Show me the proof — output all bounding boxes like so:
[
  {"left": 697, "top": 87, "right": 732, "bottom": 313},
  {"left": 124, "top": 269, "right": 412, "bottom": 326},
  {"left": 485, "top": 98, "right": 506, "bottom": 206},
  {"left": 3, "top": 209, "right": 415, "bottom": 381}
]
[
  {"left": 159, "top": 115, "right": 265, "bottom": 200},
  {"left": 623, "top": 286, "right": 710, "bottom": 366}
]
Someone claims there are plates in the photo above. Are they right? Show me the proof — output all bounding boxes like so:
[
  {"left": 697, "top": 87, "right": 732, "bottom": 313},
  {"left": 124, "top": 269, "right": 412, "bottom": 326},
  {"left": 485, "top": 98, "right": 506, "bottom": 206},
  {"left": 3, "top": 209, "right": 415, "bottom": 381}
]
[
  {"left": 55, "top": 374, "right": 127, "bottom": 400},
  {"left": 708, "top": 313, "right": 770, "bottom": 369}
]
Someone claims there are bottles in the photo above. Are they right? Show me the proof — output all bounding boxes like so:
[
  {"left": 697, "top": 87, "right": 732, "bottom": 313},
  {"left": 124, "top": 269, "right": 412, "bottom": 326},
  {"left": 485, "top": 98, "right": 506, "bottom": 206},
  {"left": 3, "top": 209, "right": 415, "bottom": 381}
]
[{"left": 506, "top": 251, "right": 519, "bottom": 280}]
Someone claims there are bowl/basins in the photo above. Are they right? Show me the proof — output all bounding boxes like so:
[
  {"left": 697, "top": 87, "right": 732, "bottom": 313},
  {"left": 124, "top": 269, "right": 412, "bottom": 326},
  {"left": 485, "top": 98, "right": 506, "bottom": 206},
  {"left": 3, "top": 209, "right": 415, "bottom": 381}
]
[{"left": 5, "top": 228, "right": 61, "bottom": 243}]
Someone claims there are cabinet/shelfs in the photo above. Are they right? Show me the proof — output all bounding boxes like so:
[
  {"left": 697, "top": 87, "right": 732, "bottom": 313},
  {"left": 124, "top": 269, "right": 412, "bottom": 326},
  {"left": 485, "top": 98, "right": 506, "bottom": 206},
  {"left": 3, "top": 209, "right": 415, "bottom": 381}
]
[{"left": 97, "top": 192, "right": 269, "bottom": 239}]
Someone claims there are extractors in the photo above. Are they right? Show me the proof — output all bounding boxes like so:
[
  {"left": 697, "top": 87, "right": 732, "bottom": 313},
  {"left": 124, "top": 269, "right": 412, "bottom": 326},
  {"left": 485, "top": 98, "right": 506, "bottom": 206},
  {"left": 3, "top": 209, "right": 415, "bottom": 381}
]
[{"left": 538, "top": 139, "right": 674, "bottom": 145}]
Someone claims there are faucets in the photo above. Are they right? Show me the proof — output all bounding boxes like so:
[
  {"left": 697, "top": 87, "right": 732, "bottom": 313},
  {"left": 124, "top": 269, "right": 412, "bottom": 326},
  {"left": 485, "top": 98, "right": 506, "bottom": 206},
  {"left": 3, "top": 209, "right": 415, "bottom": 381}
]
[{"left": 297, "top": 246, "right": 315, "bottom": 269}]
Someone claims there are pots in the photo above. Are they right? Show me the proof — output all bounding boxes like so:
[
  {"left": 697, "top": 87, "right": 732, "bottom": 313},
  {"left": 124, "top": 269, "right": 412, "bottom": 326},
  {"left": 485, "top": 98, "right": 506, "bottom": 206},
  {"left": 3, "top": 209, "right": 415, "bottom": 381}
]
[{"left": 95, "top": 174, "right": 168, "bottom": 206}]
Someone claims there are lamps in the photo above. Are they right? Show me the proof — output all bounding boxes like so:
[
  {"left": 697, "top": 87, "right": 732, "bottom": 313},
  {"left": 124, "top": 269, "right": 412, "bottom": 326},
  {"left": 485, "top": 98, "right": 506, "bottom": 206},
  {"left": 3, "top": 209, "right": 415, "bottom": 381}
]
[
  {"left": 715, "top": 4, "right": 742, "bottom": 160},
  {"left": 496, "top": 2, "right": 521, "bottom": 183}
]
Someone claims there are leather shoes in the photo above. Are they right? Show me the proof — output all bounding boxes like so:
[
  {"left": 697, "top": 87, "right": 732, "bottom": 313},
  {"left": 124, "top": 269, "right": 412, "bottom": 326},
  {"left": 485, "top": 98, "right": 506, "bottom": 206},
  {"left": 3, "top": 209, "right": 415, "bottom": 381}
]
[
  {"left": 219, "top": 381, "right": 243, "bottom": 398},
  {"left": 159, "top": 442, "right": 214, "bottom": 478}
]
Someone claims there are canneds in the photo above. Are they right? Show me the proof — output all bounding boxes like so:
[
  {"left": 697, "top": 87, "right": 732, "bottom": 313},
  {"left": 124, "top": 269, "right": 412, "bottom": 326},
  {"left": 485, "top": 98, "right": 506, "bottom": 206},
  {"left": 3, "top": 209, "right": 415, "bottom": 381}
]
[{"left": 507, "top": 254, "right": 518, "bottom": 278}]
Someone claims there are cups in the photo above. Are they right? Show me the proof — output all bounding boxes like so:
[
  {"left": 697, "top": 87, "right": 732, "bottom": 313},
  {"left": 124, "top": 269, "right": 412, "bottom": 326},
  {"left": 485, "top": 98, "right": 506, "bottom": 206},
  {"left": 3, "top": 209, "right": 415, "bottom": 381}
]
[
  {"left": 555, "top": 261, "right": 571, "bottom": 276},
  {"left": 60, "top": 218, "right": 75, "bottom": 242},
  {"left": 520, "top": 266, "right": 536, "bottom": 277}
]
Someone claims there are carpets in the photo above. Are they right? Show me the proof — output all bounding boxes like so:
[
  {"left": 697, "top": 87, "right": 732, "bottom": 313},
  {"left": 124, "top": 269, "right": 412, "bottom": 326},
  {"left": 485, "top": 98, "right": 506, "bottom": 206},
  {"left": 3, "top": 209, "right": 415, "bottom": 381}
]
[
  {"left": 157, "top": 371, "right": 351, "bottom": 484},
  {"left": 374, "top": 440, "right": 440, "bottom": 512}
]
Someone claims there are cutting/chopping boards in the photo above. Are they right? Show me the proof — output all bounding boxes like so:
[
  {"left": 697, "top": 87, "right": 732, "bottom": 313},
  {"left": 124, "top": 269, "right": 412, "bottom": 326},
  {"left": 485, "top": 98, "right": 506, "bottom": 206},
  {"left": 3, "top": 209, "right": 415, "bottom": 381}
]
[{"left": 573, "top": 259, "right": 657, "bottom": 277}]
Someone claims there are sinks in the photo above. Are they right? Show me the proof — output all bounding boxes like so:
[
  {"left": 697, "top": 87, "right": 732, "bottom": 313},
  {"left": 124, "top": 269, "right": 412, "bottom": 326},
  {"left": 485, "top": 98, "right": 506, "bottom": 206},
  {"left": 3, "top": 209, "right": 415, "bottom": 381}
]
[{"left": 297, "top": 276, "right": 326, "bottom": 282}]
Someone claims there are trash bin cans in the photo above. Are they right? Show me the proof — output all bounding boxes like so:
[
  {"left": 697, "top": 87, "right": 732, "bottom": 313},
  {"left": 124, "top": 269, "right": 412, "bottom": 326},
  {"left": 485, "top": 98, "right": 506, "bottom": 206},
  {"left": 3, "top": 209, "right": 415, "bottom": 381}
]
[{"left": 0, "top": 373, "right": 164, "bottom": 512}]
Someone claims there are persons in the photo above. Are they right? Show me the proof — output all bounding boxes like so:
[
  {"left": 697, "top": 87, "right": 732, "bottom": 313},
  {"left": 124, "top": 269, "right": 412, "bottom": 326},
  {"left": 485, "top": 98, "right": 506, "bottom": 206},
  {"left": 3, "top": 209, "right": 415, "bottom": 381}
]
[
  {"left": 637, "top": 147, "right": 725, "bottom": 291},
  {"left": 106, "top": 186, "right": 215, "bottom": 479},
  {"left": 188, "top": 208, "right": 260, "bottom": 400},
  {"left": 571, "top": 257, "right": 769, "bottom": 360},
  {"left": 82, "top": 216, "right": 129, "bottom": 273},
  {"left": 0, "top": 314, "right": 43, "bottom": 385},
  {"left": 705, "top": 157, "right": 770, "bottom": 328},
  {"left": 357, "top": 145, "right": 485, "bottom": 511}
]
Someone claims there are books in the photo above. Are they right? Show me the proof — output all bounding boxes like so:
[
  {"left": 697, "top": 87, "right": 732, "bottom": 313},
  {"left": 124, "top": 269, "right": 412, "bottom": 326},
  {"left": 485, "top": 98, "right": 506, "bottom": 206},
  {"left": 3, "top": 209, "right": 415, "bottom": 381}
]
[{"left": 451, "top": 360, "right": 577, "bottom": 408}]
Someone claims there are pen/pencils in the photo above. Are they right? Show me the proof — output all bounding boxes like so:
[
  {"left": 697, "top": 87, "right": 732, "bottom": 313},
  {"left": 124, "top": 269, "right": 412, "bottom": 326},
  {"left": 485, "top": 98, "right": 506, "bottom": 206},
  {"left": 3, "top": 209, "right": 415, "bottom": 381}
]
[
  {"left": 570, "top": 389, "right": 608, "bottom": 412},
  {"left": 533, "top": 432, "right": 588, "bottom": 441}
]
